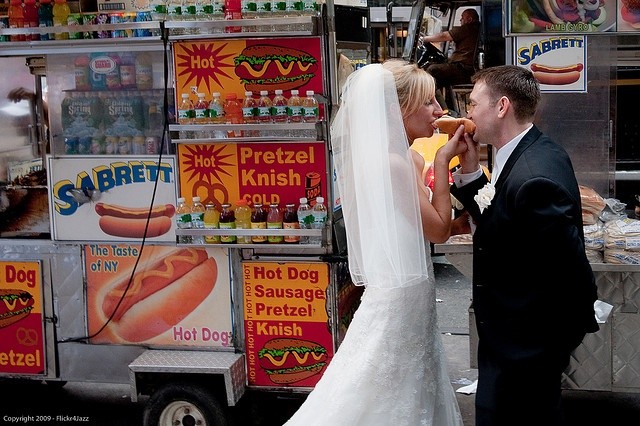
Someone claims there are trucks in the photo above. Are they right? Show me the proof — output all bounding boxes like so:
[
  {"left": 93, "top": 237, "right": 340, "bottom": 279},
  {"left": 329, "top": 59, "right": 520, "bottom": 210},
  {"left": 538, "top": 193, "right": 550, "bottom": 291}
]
[{"left": 386, "top": 1, "right": 640, "bottom": 159}]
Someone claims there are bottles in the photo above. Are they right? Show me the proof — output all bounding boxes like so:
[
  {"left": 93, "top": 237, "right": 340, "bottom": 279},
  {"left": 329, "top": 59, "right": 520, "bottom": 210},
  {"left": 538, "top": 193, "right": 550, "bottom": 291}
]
[
  {"left": 297, "top": 197, "right": 312, "bottom": 245},
  {"left": 204, "top": 203, "right": 221, "bottom": 244},
  {"left": 218, "top": 203, "right": 237, "bottom": 244},
  {"left": 299, "top": 0, "right": 318, "bottom": 33},
  {"left": 149, "top": 0, "right": 167, "bottom": 38},
  {"left": 7, "top": 0, "right": 25, "bottom": 41},
  {"left": 234, "top": 199, "right": 253, "bottom": 244},
  {"left": 181, "top": 0, "right": 196, "bottom": 35},
  {"left": 270, "top": 0, "right": 286, "bottom": 32},
  {"left": 251, "top": 203, "right": 268, "bottom": 244},
  {"left": 60, "top": 90, "right": 174, "bottom": 134},
  {"left": 134, "top": 51, "right": 154, "bottom": 90},
  {"left": 223, "top": 0, "right": 243, "bottom": 33},
  {"left": 106, "top": 52, "right": 123, "bottom": 91},
  {"left": 195, "top": 0, "right": 213, "bottom": 34},
  {"left": 175, "top": 197, "right": 192, "bottom": 244},
  {"left": 285, "top": 0, "right": 301, "bottom": 32},
  {"left": 88, "top": 52, "right": 109, "bottom": 91},
  {"left": 208, "top": 92, "right": 227, "bottom": 138},
  {"left": 242, "top": 91, "right": 257, "bottom": 137},
  {"left": 255, "top": 0, "right": 271, "bottom": 32},
  {"left": 266, "top": 204, "right": 284, "bottom": 244},
  {"left": 272, "top": 89, "right": 287, "bottom": 137},
  {"left": 302, "top": 90, "right": 319, "bottom": 137},
  {"left": 212, "top": 0, "right": 224, "bottom": 34},
  {"left": 166, "top": 0, "right": 181, "bottom": 36},
  {"left": 74, "top": 53, "right": 92, "bottom": 91},
  {"left": 22, "top": 0, "right": 39, "bottom": 41},
  {"left": 178, "top": 93, "right": 195, "bottom": 139},
  {"left": 282, "top": 203, "right": 300, "bottom": 244},
  {"left": 287, "top": 90, "right": 302, "bottom": 137},
  {"left": 37, "top": 0, "right": 55, "bottom": 41},
  {"left": 241, "top": 0, "right": 256, "bottom": 32},
  {"left": 119, "top": 51, "right": 137, "bottom": 91},
  {"left": 194, "top": 93, "right": 210, "bottom": 138},
  {"left": 223, "top": 92, "right": 244, "bottom": 138},
  {"left": 190, "top": 196, "right": 205, "bottom": 244},
  {"left": 52, "top": 0, "right": 71, "bottom": 40},
  {"left": 309, "top": 196, "right": 328, "bottom": 243},
  {"left": 257, "top": 90, "right": 272, "bottom": 137}
]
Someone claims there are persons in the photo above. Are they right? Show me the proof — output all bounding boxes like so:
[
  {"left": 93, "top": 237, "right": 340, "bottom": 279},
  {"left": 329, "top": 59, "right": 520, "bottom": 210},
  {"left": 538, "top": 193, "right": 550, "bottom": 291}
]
[
  {"left": 1, "top": 86, "right": 50, "bottom": 154},
  {"left": 284, "top": 57, "right": 472, "bottom": 426},
  {"left": 450, "top": 65, "right": 600, "bottom": 426},
  {"left": 418, "top": 8, "right": 480, "bottom": 115}
]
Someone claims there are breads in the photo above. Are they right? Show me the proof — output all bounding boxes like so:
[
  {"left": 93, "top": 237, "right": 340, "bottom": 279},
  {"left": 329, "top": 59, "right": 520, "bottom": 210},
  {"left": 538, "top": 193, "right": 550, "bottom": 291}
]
[
  {"left": 582, "top": 213, "right": 598, "bottom": 225},
  {"left": 578, "top": 185, "right": 606, "bottom": 213}
]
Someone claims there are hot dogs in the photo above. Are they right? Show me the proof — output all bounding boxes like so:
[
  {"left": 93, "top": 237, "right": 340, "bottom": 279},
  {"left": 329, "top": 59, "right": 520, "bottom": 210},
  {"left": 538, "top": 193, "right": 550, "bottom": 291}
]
[
  {"left": 432, "top": 115, "right": 475, "bottom": 135},
  {"left": 528, "top": 61, "right": 583, "bottom": 85},
  {"left": 234, "top": 42, "right": 318, "bottom": 96},
  {"left": 1, "top": 288, "right": 35, "bottom": 327},
  {"left": 94, "top": 200, "right": 175, "bottom": 239},
  {"left": 97, "top": 247, "right": 217, "bottom": 343},
  {"left": 258, "top": 338, "right": 329, "bottom": 384}
]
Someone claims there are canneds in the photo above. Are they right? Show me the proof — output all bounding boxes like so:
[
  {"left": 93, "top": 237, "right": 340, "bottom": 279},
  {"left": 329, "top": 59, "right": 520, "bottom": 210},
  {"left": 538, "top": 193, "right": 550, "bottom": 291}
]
[
  {"left": 109, "top": 13, "right": 123, "bottom": 38},
  {"left": 135, "top": 11, "right": 151, "bottom": 37},
  {"left": 94, "top": 12, "right": 109, "bottom": 38},
  {"left": 105, "top": 136, "right": 118, "bottom": 154},
  {"left": 80, "top": 138, "right": 92, "bottom": 155},
  {"left": 118, "top": 134, "right": 131, "bottom": 154},
  {"left": 66, "top": 13, "right": 83, "bottom": 40},
  {"left": 145, "top": 135, "right": 161, "bottom": 155},
  {"left": 132, "top": 133, "right": 146, "bottom": 155},
  {"left": 65, "top": 136, "right": 79, "bottom": 154},
  {"left": 121, "top": 13, "right": 136, "bottom": 38},
  {"left": 91, "top": 138, "right": 104, "bottom": 155},
  {"left": 81, "top": 14, "right": 96, "bottom": 38},
  {"left": 304, "top": 171, "right": 322, "bottom": 207}
]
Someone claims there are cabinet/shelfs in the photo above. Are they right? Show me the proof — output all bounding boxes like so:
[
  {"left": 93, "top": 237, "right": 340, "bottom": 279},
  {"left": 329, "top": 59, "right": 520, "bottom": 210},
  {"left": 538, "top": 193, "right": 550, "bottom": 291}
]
[
  {"left": 0, "top": 21, "right": 165, "bottom": 56},
  {"left": 164, "top": 15, "right": 327, "bottom": 249},
  {"left": 60, "top": 82, "right": 173, "bottom": 157}
]
